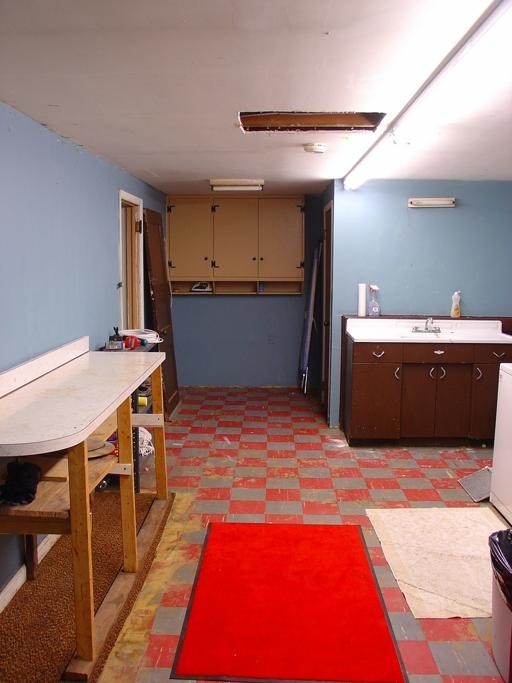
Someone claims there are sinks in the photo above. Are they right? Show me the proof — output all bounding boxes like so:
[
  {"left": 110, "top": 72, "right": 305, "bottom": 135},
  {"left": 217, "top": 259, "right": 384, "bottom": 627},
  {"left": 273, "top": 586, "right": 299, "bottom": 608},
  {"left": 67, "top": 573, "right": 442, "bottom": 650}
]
[{"left": 344, "top": 317, "right": 512, "bottom": 344}]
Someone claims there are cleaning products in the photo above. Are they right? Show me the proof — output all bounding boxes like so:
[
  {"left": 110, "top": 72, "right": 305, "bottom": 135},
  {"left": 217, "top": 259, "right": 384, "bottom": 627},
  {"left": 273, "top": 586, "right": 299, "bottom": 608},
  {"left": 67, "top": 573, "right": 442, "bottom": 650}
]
[{"left": 367, "top": 284, "right": 382, "bottom": 317}]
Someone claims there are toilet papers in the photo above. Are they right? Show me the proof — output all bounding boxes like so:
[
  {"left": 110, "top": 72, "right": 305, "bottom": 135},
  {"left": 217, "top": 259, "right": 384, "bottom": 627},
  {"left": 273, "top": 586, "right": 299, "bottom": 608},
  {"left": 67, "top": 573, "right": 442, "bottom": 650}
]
[{"left": 356, "top": 283, "right": 366, "bottom": 316}]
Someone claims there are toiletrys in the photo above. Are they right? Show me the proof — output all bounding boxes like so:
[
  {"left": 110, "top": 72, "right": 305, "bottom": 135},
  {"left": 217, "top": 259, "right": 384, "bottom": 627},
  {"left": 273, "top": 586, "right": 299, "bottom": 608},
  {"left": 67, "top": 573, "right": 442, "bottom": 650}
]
[{"left": 450, "top": 290, "right": 461, "bottom": 318}]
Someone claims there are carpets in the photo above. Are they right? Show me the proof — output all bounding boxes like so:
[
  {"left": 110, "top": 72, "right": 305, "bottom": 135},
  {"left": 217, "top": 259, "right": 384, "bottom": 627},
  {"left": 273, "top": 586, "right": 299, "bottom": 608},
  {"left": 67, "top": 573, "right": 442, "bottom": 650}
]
[
  {"left": 169, "top": 519, "right": 410, "bottom": 682},
  {"left": 365, "top": 508, "right": 511, "bottom": 620},
  {"left": 0, "top": 488, "right": 175, "bottom": 683}
]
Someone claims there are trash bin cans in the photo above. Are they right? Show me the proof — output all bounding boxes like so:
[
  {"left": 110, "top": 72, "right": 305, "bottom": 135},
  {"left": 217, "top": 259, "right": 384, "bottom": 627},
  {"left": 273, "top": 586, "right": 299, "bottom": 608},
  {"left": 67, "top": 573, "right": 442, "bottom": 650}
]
[{"left": 490, "top": 530, "right": 512, "bottom": 683}]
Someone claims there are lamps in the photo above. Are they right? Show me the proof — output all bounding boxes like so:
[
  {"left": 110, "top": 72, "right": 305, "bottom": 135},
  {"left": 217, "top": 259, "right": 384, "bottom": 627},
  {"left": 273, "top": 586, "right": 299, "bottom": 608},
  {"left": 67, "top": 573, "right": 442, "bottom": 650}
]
[
  {"left": 407, "top": 197, "right": 457, "bottom": 208},
  {"left": 208, "top": 178, "right": 264, "bottom": 192}
]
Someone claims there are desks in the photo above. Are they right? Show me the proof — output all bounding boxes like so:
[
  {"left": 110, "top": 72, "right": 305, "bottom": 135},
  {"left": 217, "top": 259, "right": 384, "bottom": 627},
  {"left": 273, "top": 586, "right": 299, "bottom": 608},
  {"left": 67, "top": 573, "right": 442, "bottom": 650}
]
[{"left": 0, "top": 333, "right": 176, "bottom": 683}]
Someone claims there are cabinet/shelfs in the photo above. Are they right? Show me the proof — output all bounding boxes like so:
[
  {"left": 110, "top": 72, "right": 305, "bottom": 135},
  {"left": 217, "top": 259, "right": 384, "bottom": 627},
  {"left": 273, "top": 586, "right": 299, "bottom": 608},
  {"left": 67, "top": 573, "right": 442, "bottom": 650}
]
[
  {"left": 96, "top": 342, "right": 160, "bottom": 471},
  {"left": 488, "top": 363, "right": 512, "bottom": 526},
  {"left": 339, "top": 333, "right": 403, "bottom": 445},
  {"left": 402, "top": 344, "right": 475, "bottom": 447},
  {"left": 211, "top": 196, "right": 306, "bottom": 294},
  {"left": 166, "top": 195, "right": 214, "bottom": 294},
  {"left": 467, "top": 344, "right": 512, "bottom": 446}
]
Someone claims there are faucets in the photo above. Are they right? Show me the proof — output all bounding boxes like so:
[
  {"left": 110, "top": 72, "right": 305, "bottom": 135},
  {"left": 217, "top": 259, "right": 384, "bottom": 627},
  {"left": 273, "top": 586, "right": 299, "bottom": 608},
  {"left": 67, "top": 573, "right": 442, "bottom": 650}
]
[{"left": 424, "top": 316, "right": 433, "bottom": 330}]
